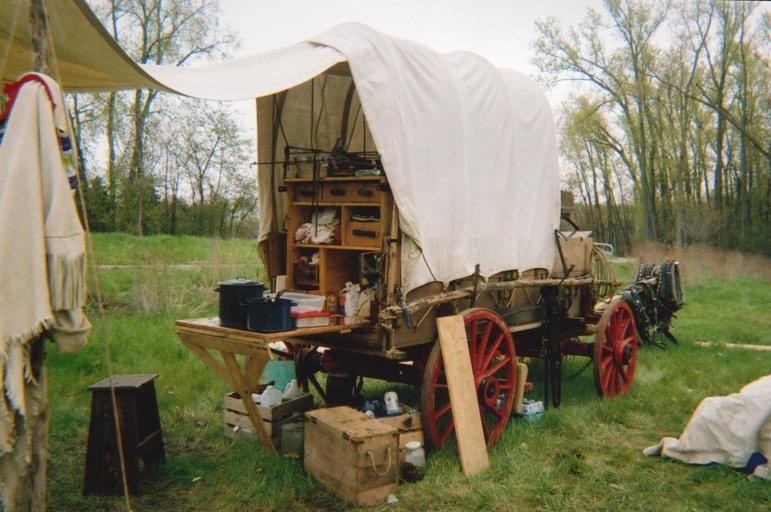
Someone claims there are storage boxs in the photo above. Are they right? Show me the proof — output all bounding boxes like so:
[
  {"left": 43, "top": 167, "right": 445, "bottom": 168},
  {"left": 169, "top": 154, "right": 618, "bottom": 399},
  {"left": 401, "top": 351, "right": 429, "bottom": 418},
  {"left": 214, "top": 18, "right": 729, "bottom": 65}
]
[
  {"left": 224, "top": 385, "right": 312, "bottom": 449},
  {"left": 552, "top": 272, "right": 593, "bottom": 316},
  {"left": 303, "top": 405, "right": 400, "bottom": 507},
  {"left": 552, "top": 230, "right": 594, "bottom": 272},
  {"left": 360, "top": 403, "right": 425, "bottom": 467}
]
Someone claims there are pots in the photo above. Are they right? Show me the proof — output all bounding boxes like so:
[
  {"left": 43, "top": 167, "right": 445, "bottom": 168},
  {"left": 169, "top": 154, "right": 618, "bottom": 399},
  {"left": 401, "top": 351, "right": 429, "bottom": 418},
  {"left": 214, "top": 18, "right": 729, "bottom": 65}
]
[
  {"left": 214, "top": 275, "right": 269, "bottom": 328},
  {"left": 239, "top": 296, "right": 297, "bottom": 333}
]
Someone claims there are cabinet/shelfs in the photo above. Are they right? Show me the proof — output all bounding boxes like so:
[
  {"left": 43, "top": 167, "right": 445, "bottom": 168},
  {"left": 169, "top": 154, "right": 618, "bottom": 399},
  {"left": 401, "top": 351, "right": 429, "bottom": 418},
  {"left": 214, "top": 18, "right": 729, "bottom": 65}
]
[{"left": 282, "top": 176, "right": 390, "bottom": 312}]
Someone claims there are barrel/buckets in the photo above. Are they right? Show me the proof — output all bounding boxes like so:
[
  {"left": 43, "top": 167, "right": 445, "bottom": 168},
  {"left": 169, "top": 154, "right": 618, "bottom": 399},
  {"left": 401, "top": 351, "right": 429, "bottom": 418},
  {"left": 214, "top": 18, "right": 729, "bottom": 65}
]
[
  {"left": 282, "top": 379, "right": 303, "bottom": 402},
  {"left": 262, "top": 384, "right": 281, "bottom": 406}
]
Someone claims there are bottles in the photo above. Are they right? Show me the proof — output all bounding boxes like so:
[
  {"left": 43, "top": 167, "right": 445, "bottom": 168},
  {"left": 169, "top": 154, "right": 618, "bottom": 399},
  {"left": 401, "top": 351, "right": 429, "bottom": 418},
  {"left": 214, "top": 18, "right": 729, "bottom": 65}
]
[
  {"left": 496, "top": 394, "right": 544, "bottom": 421},
  {"left": 403, "top": 441, "right": 427, "bottom": 477}
]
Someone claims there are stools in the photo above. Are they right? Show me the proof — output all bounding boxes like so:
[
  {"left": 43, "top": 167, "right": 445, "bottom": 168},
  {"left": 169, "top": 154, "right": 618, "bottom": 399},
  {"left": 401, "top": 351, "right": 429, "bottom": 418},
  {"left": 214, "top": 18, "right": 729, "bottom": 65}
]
[{"left": 82, "top": 373, "right": 165, "bottom": 496}]
